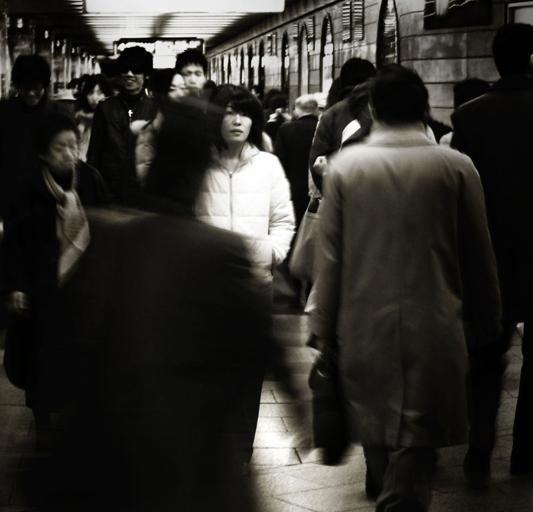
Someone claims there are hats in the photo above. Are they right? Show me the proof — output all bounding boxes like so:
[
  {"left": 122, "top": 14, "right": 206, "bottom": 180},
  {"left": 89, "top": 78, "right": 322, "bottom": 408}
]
[{"left": 9, "top": 54, "right": 51, "bottom": 90}]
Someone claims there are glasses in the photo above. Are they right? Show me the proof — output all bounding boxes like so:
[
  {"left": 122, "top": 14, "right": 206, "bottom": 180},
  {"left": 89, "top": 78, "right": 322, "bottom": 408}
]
[{"left": 119, "top": 65, "right": 143, "bottom": 74}]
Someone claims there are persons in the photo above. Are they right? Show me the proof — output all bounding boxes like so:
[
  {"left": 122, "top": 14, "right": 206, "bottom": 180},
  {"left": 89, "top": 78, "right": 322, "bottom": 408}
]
[{"left": 1, "top": 20, "right": 533, "bottom": 511}]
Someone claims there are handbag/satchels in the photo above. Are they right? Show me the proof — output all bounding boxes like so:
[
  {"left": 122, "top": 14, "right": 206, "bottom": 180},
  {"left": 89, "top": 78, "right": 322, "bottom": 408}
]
[
  {"left": 289, "top": 191, "right": 323, "bottom": 280},
  {"left": 308, "top": 354, "right": 357, "bottom": 464}
]
